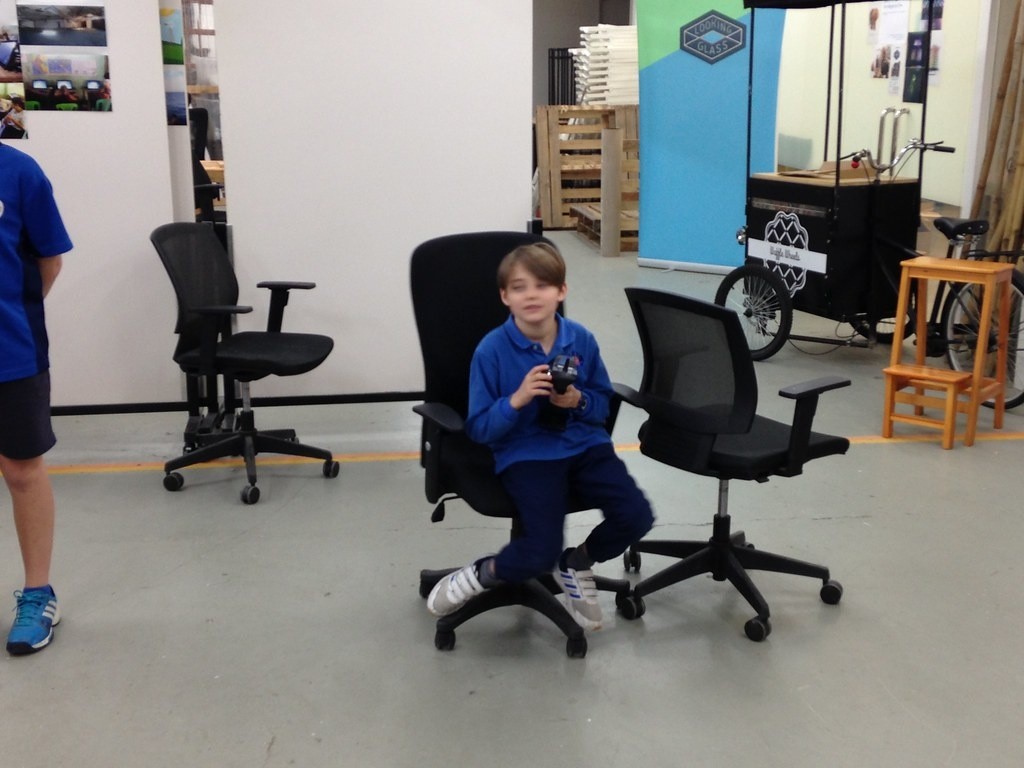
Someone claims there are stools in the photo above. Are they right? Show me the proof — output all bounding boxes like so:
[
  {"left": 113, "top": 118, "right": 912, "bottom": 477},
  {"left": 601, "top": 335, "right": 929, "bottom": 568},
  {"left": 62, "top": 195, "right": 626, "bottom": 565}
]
[{"left": 883, "top": 255, "right": 1014, "bottom": 448}]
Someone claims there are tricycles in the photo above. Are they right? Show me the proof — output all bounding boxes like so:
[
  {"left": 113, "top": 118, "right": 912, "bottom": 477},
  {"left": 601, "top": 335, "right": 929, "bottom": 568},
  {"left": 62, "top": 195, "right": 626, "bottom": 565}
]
[{"left": 715, "top": 1, "right": 1024, "bottom": 409}]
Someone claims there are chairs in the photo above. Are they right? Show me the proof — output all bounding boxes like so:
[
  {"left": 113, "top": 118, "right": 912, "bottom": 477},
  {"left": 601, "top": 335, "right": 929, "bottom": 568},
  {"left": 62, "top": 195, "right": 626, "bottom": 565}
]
[
  {"left": 624, "top": 287, "right": 851, "bottom": 641},
  {"left": 409, "top": 232, "right": 652, "bottom": 659},
  {"left": 150, "top": 222, "right": 341, "bottom": 505}
]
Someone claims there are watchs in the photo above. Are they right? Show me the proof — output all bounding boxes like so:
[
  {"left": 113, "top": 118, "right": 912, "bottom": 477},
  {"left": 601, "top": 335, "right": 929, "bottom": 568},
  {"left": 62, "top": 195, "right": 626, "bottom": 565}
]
[{"left": 576, "top": 393, "right": 587, "bottom": 412}]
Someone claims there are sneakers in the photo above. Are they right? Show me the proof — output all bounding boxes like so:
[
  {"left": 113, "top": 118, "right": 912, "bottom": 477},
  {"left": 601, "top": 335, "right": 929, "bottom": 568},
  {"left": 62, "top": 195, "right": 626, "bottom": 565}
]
[
  {"left": 5, "top": 584, "right": 61, "bottom": 657},
  {"left": 550, "top": 547, "right": 603, "bottom": 633},
  {"left": 427, "top": 556, "right": 497, "bottom": 618}
]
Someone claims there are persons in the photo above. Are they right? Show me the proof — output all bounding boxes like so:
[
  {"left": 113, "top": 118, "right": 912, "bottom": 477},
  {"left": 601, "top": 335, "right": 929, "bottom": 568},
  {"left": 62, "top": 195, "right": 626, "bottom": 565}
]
[
  {"left": 426, "top": 241, "right": 654, "bottom": 632},
  {"left": 0, "top": 72, "right": 111, "bottom": 139},
  {"left": 0, "top": 141, "right": 74, "bottom": 653}
]
